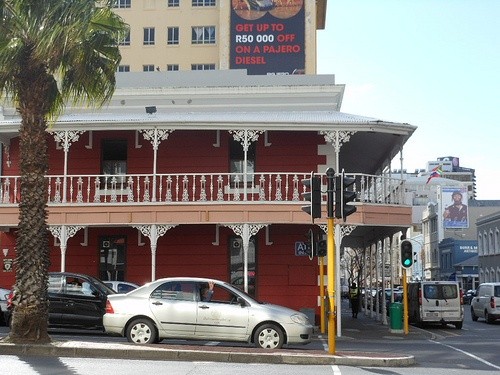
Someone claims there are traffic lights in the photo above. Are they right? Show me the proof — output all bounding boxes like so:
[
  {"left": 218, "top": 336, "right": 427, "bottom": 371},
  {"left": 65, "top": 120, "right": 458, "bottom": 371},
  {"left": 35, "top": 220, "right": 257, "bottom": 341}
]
[
  {"left": 334, "top": 168, "right": 356, "bottom": 221},
  {"left": 301, "top": 171, "right": 322, "bottom": 225},
  {"left": 400, "top": 238, "right": 414, "bottom": 269}
]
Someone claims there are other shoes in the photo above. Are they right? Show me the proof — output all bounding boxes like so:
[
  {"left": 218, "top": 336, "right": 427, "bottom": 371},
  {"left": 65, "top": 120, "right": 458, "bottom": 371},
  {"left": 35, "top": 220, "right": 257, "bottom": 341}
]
[
  {"left": 355, "top": 316, "right": 357, "bottom": 319},
  {"left": 352, "top": 315, "right": 354, "bottom": 318}
]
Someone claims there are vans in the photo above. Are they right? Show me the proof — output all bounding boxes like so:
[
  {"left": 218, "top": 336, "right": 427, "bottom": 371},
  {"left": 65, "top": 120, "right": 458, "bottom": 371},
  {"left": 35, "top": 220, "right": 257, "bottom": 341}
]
[{"left": 406, "top": 279, "right": 464, "bottom": 329}]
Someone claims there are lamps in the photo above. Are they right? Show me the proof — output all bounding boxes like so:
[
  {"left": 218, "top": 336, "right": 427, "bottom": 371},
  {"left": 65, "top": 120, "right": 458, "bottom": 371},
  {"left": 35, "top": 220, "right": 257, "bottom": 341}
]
[{"left": 145, "top": 105, "right": 157, "bottom": 114}]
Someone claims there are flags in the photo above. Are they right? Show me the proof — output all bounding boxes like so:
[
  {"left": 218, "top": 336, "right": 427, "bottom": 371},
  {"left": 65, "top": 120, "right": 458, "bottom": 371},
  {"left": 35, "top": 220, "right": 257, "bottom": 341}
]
[{"left": 426, "top": 164, "right": 442, "bottom": 184}]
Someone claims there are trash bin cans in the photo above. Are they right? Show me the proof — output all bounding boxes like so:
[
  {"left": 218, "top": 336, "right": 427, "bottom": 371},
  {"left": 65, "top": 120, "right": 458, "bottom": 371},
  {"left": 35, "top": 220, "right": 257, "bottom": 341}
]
[{"left": 390, "top": 302, "right": 404, "bottom": 329}]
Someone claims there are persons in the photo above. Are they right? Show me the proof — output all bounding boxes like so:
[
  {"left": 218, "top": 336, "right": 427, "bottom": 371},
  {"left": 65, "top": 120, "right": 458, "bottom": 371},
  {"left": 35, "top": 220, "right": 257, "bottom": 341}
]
[
  {"left": 349, "top": 283, "right": 365, "bottom": 318},
  {"left": 443, "top": 191, "right": 467, "bottom": 224},
  {"left": 70, "top": 281, "right": 81, "bottom": 295},
  {"left": 199, "top": 281, "right": 215, "bottom": 302}
]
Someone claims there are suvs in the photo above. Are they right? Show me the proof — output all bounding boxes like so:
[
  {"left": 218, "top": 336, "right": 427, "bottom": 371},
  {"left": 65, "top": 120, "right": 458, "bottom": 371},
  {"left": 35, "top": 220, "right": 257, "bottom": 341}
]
[{"left": 470, "top": 281, "right": 500, "bottom": 323}]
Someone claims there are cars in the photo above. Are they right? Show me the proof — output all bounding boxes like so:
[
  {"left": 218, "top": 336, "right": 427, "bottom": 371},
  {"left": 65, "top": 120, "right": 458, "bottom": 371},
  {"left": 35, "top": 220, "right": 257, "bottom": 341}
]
[
  {"left": 361, "top": 287, "right": 403, "bottom": 314},
  {"left": 103, "top": 280, "right": 138, "bottom": 294},
  {"left": 7, "top": 271, "right": 117, "bottom": 331},
  {"left": 101, "top": 276, "right": 315, "bottom": 349}
]
